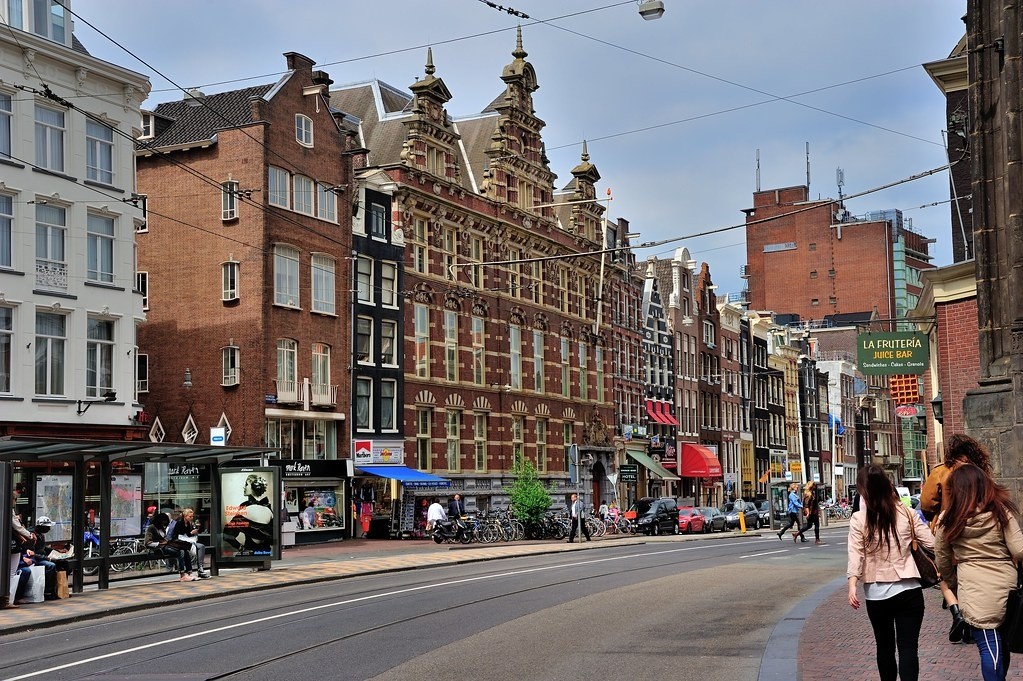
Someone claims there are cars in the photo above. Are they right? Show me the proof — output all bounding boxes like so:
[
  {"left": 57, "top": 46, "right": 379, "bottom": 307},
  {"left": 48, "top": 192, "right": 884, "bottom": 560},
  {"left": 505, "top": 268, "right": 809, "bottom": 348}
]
[
  {"left": 753, "top": 500, "right": 782, "bottom": 529},
  {"left": 697, "top": 507, "right": 728, "bottom": 533},
  {"left": 677, "top": 506, "right": 708, "bottom": 534},
  {"left": 719, "top": 502, "right": 760, "bottom": 528}
]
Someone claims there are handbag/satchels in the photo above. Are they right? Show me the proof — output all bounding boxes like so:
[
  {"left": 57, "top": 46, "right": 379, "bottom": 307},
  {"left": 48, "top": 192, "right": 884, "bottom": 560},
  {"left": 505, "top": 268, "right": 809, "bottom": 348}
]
[
  {"left": 178, "top": 534, "right": 197, "bottom": 556},
  {"left": 10, "top": 527, "right": 21, "bottom": 554},
  {"left": 9, "top": 570, "right": 22, "bottom": 604},
  {"left": 424, "top": 523, "right": 432, "bottom": 531},
  {"left": 1003, "top": 558, "right": 1023, "bottom": 654},
  {"left": 167, "top": 539, "right": 192, "bottom": 551},
  {"left": 24, "top": 563, "right": 45, "bottom": 602},
  {"left": 901, "top": 503, "right": 943, "bottom": 588}
]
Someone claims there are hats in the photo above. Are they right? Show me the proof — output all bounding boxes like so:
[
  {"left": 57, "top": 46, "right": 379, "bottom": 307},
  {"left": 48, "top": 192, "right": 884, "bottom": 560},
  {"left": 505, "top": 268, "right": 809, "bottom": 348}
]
[
  {"left": 35, "top": 515, "right": 56, "bottom": 527},
  {"left": 146, "top": 506, "right": 156, "bottom": 514}
]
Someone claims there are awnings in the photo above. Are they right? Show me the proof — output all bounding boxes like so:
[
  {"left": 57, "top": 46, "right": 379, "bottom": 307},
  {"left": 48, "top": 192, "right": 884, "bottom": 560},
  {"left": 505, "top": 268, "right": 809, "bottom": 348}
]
[
  {"left": 357, "top": 466, "right": 451, "bottom": 487},
  {"left": 627, "top": 451, "right": 681, "bottom": 480},
  {"left": 648, "top": 411, "right": 680, "bottom": 426},
  {"left": 681, "top": 443, "right": 722, "bottom": 478}
]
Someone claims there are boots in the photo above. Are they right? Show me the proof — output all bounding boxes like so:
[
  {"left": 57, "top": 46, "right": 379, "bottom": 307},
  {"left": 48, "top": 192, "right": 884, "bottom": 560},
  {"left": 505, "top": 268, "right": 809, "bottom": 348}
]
[
  {"left": 949, "top": 604, "right": 967, "bottom": 642},
  {"left": 963, "top": 622, "right": 976, "bottom": 644}
]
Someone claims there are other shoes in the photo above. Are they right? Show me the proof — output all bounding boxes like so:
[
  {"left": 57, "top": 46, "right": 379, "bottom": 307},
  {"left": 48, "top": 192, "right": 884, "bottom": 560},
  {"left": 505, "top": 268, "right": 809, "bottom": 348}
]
[
  {"left": 198, "top": 573, "right": 213, "bottom": 580},
  {"left": 792, "top": 531, "right": 800, "bottom": 543},
  {"left": 567, "top": 540, "right": 574, "bottom": 543},
  {"left": 815, "top": 540, "right": 826, "bottom": 545},
  {"left": 801, "top": 538, "right": 809, "bottom": 542},
  {"left": 5, "top": 604, "right": 20, "bottom": 609},
  {"left": 44, "top": 593, "right": 61, "bottom": 600},
  {"left": 942, "top": 588, "right": 957, "bottom": 610},
  {"left": 180, "top": 574, "right": 194, "bottom": 582},
  {"left": 586, "top": 539, "right": 591, "bottom": 541},
  {"left": 190, "top": 574, "right": 201, "bottom": 581},
  {"left": 777, "top": 531, "right": 782, "bottom": 540}
]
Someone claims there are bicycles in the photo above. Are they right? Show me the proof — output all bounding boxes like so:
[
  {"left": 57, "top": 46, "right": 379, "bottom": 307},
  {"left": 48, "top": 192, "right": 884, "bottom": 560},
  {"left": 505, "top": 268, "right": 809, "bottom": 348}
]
[
  {"left": 431, "top": 503, "right": 636, "bottom": 544},
  {"left": 819, "top": 501, "right": 852, "bottom": 520},
  {"left": 79, "top": 520, "right": 171, "bottom": 577}
]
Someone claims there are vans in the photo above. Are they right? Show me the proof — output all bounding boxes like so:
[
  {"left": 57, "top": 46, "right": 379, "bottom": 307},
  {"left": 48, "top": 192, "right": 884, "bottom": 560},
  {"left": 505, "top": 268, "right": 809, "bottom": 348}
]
[{"left": 627, "top": 497, "right": 680, "bottom": 536}]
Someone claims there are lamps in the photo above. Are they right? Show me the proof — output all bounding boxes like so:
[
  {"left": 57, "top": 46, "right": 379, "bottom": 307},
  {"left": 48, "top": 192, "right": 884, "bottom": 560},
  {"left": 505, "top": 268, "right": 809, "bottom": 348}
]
[{"left": 930, "top": 390, "right": 944, "bottom": 424}]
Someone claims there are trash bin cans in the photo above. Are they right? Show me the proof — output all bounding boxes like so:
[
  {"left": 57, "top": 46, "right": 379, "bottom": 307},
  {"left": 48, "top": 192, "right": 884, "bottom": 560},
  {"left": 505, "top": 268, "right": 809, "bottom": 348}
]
[{"left": 820, "top": 509, "right": 828, "bottom": 526}]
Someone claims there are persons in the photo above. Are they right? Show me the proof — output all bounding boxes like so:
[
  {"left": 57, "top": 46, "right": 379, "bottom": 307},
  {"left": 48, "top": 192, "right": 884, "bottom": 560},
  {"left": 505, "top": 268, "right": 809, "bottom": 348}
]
[
  {"left": 919, "top": 433, "right": 1023, "bottom": 681},
  {"left": 792, "top": 480, "right": 826, "bottom": 545},
  {"left": 8, "top": 491, "right": 38, "bottom": 608},
  {"left": 776, "top": 483, "right": 809, "bottom": 543},
  {"left": 25, "top": 515, "right": 72, "bottom": 599},
  {"left": 567, "top": 494, "right": 591, "bottom": 542},
  {"left": 598, "top": 500, "right": 608, "bottom": 518},
  {"left": 145, "top": 513, "right": 193, "bottom": 581},
  {"left": 846, "top": 463, "right": 936, "bottom": 681},
  {"left": 16, "top": 550, "right": 36, "bottom": 603},
  {"left": 171, "top": 508, "right": 211, "bottom": 580},
  {"left": 223, "top": 474, "right": 273, "bottom": 551},
  {"left": 827, "top": 495, "right": 833, "bottom": 504},
  {"left": 449, "top": 494, "right": 463, "bottom": 515},
  {"left": 427, "top": 499, "right": 447, "bottom": 526}
]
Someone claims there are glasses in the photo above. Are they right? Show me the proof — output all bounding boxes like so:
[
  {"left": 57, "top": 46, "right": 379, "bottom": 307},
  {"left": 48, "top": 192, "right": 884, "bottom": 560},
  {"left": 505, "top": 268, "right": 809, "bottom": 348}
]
[{"left": 13, "top": 497, "right": 18, "bottom": 502}]
